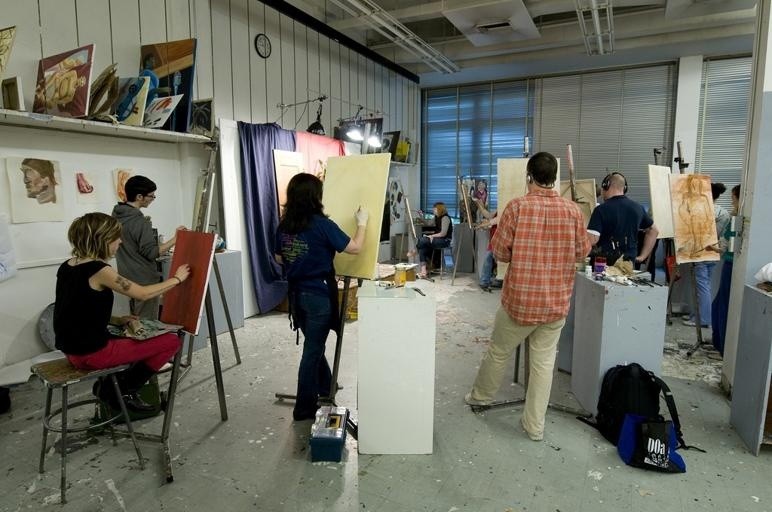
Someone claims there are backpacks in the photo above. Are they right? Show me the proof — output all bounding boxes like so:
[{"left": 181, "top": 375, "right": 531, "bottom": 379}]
[{"left": 596, "top": 363, "right": 687, "bottom": 449}]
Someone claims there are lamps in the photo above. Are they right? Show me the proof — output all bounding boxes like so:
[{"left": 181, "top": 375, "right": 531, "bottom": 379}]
[
  {"left": 573, "top": 0, "right": 616, "bottom": 57},
  {"left": 337, "top": 103, "right": 368, "bottom": 145},
  {"left": 332, "top": 1, "right": 461, "bottom": 75},
  {"left": 277, "top": 96, "right": 330, "bottom": 136},
  {"left": 368, "top": 110, "right": 384, "bottom": 149}
]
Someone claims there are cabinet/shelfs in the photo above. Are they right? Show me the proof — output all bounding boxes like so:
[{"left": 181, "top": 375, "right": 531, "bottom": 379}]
[{"left": 729, "top": 285, "right": 772, "bottom": 456}]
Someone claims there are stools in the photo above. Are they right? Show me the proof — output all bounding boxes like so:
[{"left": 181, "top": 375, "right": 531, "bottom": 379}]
[
  {"left": 428, "top": 248, "right": 453, "bottom": 280},
  {"left": 30, "top": 357, "right": 145, "bottom": 505}
]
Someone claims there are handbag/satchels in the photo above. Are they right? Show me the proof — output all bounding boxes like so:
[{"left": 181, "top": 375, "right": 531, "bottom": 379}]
[{"left": 617, "top": 413, "right": 686, "bottom": 473}]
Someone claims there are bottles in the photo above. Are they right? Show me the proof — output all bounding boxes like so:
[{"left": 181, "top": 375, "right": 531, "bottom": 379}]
[
  {"left": 394, "top": 266, "right": 406, "bottom": 287},
  {"left": 585, "top": 266, "right": 592, "bottom": 280}
]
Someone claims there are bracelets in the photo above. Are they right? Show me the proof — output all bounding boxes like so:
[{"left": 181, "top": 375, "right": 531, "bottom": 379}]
[
  {"left": 712, "top": 246, "right": 715, "bottom": 252},
  {"left": 170, "top": 275, "right": 182, "bottom": 286}
]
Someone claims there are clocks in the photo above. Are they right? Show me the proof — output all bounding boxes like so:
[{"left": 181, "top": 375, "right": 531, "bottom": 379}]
[{"left": 254, "top": 33, "right": 272, "bottom": 59}]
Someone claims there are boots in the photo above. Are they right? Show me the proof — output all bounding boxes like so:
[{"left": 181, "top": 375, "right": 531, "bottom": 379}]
[{"left": 120, "top": 361, "right": 156, "bottom": 412}]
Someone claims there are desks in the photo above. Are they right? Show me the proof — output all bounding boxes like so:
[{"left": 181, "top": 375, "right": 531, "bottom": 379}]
[{"left": 414, "top": 224, "right": 437, "bottom": 242}]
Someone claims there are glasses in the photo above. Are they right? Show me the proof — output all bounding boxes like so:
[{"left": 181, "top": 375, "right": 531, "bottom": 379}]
[{"left": 145, "top": 196, "right": 156, "bottom": 198}]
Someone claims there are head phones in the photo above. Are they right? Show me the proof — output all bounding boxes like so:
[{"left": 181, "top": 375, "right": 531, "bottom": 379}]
[
  {"left": 601, "top": 172, "right": 628, "bottom": 194},
  {"left": 525, "top": 172, "right": 555, "bottom": 190}
]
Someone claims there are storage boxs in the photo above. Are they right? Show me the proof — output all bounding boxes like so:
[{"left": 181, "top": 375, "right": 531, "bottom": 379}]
[{"left": 310, "top": 406, "right": 350, "bottom": 463}]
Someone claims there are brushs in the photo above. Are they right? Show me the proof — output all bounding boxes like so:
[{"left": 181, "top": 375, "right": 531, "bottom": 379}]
[{"left": 412, "top": 286, "right": 426, "bottom": 297}]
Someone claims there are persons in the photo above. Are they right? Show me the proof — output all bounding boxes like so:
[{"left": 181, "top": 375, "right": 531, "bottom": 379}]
[
  {"left": 128, "top": 83, "right": 139, "bottom": 98},
  {"left": 681, "top": 180, "right": 728, "bottom": 330},
  {"left": 474, "top": 179, "right": 488, "bottom": 205},
  {"left": 637, "top": 207, "right": 660, "bottom": 284},
  {"left": 474, "top": 198, "right": 499, "bottom": 292},
  {"left": 53, "top": 210, "right": 191, "bottom": 417},
  {"left": 699, "top": 185, "right": 741, "bottom": 361},
  {"left": 139, "top": 53, "right": 172, "bottom": 104},
  {"left": 585, "top": 172, "right": 660, "bottom": 271},
  {"left": 34, "top": 69, "right": 87, "bottom": 108},
  {"left": 464, "top": 152, "right": 592, "bottom": 443},
  {"left": 416, "top": 201, "right": 453, "bottom": 278},
  {"left": 274, "top": 171, "right": 371, "bottom": 421},
  {"left": 108, "top": 174, "right": 190, "bottom": 320},
  {"left": 20, "top": 158, "right": 58, "bottom": 206}
]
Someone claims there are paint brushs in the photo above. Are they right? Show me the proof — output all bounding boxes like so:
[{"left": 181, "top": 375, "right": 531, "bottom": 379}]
[
  {"left": 643, "top": 278, "right": 662, "bottom": 286},
  {"left": 638, "top": 280, "right": 654, "bottom": 287},
  {"left": 628, "top": 278, "right": 638, "bottom": 285},
  {"left": 695, "top": 242, "right": 719, "bottom": 254}
]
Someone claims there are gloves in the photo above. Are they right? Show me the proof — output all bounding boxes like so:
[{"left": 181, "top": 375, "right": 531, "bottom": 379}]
[{"left": 355, "top": 208, "right": 369, "bottom": 225}]
